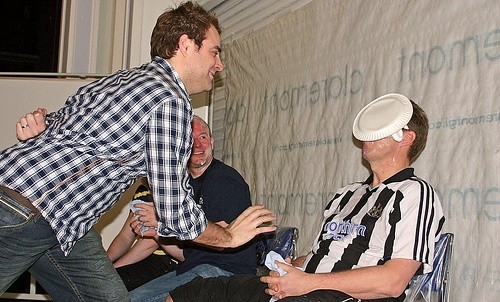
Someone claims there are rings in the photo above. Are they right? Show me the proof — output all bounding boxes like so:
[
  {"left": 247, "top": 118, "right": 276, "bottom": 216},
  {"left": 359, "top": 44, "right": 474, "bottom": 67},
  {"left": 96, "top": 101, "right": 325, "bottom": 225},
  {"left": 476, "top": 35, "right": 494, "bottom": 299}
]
[{"left": 21, "top": 125, "right": 27, "bottom": 128}]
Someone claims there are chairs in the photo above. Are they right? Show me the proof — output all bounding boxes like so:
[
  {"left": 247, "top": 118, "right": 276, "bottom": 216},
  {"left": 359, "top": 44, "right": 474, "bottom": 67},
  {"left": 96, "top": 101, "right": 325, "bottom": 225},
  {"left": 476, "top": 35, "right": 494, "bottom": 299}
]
[
  {"left": 257, "top": 224, "right": 299, "bottom": 274},
  {"left": 402, "top": 232, "right": 454, "bottom": 302}
]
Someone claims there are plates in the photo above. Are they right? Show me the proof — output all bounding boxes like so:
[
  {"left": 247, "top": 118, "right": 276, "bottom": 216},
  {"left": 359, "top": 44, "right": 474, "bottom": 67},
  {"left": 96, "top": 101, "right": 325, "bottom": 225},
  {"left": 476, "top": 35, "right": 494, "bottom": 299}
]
[{"left": 352, "top": 93, "right": 413, "bottom": 141}]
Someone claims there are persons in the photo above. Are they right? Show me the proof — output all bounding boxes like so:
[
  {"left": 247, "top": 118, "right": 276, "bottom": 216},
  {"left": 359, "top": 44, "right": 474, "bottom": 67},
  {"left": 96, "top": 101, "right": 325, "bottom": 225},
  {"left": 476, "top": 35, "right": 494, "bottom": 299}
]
[
  {"left": 130, "top": 116, "right": 256, "bottom": 302},
  {"left": 107, "top": 184, "right": 174, "bottom": 292},
  {"left": 165, "top": 99, "right": 446, "bottom": 302},
  {"left": 0, "top": 0, "right": 278, "bottom": 302}
]
